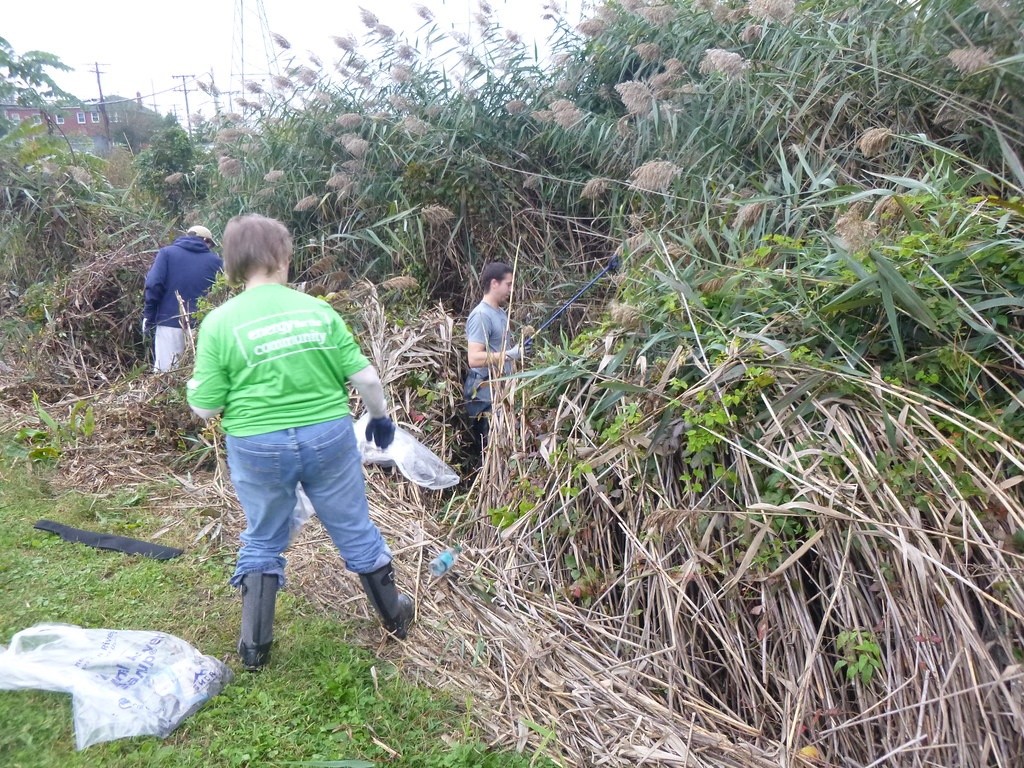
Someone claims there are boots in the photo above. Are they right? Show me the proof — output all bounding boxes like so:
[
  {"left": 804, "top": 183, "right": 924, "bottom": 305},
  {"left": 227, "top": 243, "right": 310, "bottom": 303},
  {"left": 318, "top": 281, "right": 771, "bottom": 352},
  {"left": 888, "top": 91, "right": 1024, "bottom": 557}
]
[
  {"left": 358, "top": 562, "right": 411, "bottom": 641},
  {"left": 236, "top": 572, "right": 279, "bottom": 665}
]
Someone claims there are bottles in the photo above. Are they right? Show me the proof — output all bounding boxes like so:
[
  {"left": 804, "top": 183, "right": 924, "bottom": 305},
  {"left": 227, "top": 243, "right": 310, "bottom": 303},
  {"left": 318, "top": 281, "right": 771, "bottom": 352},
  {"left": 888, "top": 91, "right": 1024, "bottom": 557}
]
[{"left": 429, "top": 546, "right": 462, "bottom": 576}]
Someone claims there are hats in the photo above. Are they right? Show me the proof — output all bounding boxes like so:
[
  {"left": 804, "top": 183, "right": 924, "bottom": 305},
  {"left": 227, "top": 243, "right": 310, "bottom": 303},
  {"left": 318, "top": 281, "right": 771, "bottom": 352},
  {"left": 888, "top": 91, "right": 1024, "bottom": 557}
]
[{"left": 186, "top": 226, "right": 219, "bottom": 247}]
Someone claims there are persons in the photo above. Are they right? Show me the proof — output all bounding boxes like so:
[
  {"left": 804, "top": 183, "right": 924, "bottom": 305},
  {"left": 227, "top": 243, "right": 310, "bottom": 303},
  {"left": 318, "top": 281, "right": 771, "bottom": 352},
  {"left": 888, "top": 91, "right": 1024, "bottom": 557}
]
[
  {"left": 142, "top": 225, "right": 225, "bottom": 373},
  {"left": 186, "top": 214, "right": 415, "bottom": 672},
  {"left": 462, "top": 262, "right": 532, "bottom": 488}
]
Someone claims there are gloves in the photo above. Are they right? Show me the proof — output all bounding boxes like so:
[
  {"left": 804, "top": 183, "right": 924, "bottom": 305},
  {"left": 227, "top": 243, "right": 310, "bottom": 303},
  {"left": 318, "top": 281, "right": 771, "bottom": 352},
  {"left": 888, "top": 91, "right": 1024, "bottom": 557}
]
[
  {"left": 142, "top": 318, "right": 151, "bottom": 338},
  {"left": 365, "top": 416, "right": 396, "bottom": 449},
  {"left": 506, "top": 343, "right": 531, "bottom": 361}
]
